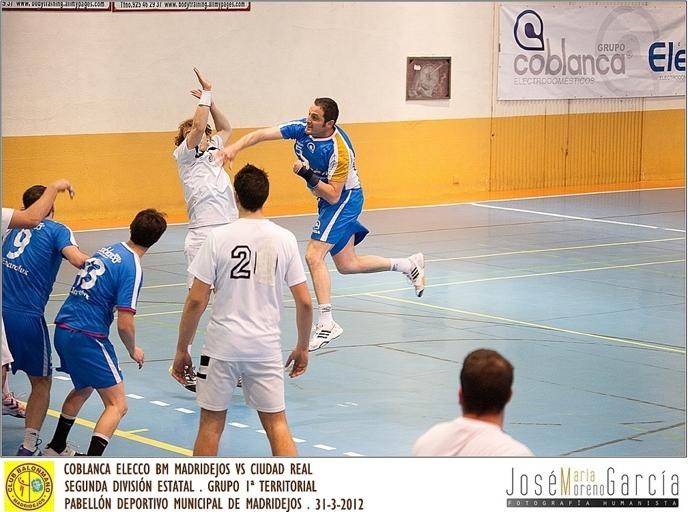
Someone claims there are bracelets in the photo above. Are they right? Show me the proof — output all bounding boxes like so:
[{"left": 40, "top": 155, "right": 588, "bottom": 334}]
[{"left": 199, "top": 89, "right": 213, "bottom": 106}]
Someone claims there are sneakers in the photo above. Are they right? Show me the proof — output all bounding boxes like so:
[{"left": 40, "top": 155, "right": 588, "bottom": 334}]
[
  {"left": 402, "top": 253, "right": 425, "bottom": 297},
  {"left": 1, "top": 391, "right": 27, "bottom": 418},
  {"left": 17, "top": 444, "right": 42, "bottom": 456},
  {"left": 169, "top": 364, "right": 197, "bottom": 392},
  {"left": 43, "top": 444, "right": 87, "bottom": 456},
  {"left": 309, "top": 320, "right": 343, "bottom": 351}
]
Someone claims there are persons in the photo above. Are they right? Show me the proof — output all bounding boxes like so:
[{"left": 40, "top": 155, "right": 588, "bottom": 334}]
[
  {"left": 410, "top": 348, "right": 537, "bottom": 457},
  {"left": 2, "top": 184, "right": 94, "bottom": 457},
  {"left": 217, "top": 97, "right": 426, "bottom": 352},
  {"left": 36, "top": 208, "right": 167, "bottom": 457},
  {"left": 2, "top": 180, "right": 76, "bottom": 418},
  {"left": 170, "top": 65, "right": 240, "bottom": 393},
  {"left": 170, "top": 161, "right": 314, "bottom": 457}
]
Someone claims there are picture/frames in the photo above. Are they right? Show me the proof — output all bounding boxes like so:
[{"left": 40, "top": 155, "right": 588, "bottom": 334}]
[{"left": 406, "top": 56, "right": 452, "bottom": 100}]
